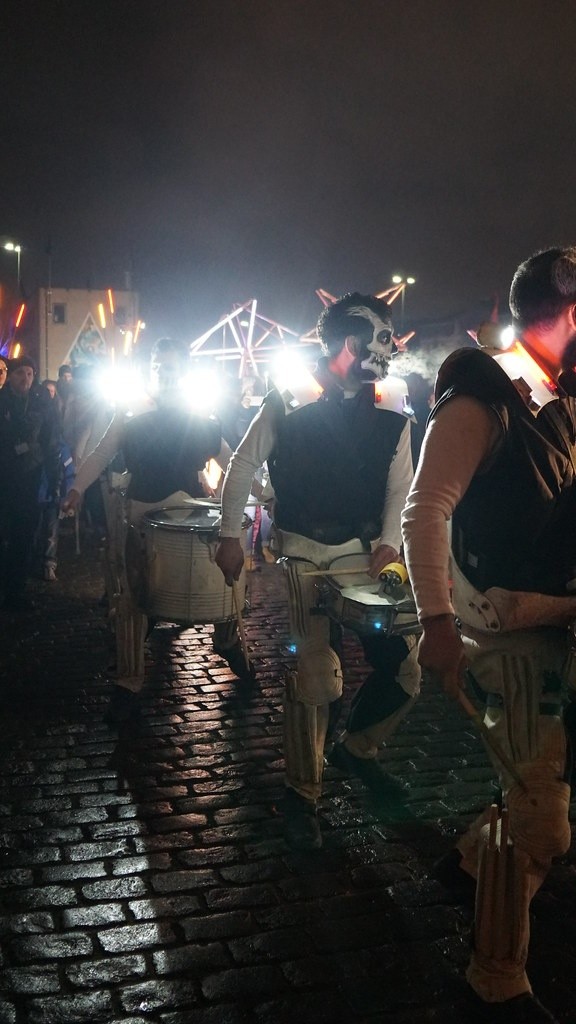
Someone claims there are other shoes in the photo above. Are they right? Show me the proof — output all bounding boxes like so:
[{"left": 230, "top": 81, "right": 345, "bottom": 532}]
[
  {"left": 460, "top": 967, "right": 561, "bottom": 1024},
  {"left": 103, "top": 682, "right": 142, "bottom": 723},
  {"left": 330, "top": 740, "right": 416, "bottom": 801},
  {"left": 210, "top": 641, "right": 258, "bottom": 679},
  {"left": 44, "top": 559, "right": 58, "bottom": 581},
  {"left": 273, "top": 791, "right": 324, "bottom": 856},
  {"left": 435, "top": 848, "right": 476, "bottom": 908}
]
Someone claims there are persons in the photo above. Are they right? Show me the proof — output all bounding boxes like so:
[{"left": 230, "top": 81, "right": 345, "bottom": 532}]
[
  {"left": 58, "top": 337, "right": 259, "bottom": 708},
  {"left": 401, "top": 244, "right": 574, "bottom": 1021},
  {"left": 213, "top": 290, "right": 418, "bottom": 852},
  {"left": 0, "top": 338, "right": 440, "bottom": 577}
]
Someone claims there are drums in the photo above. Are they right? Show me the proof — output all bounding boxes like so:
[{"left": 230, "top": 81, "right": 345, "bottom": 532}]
[
  {"left": 136, "top": 507, "right": 253, "bottom": 624},
  {"left": 322, "top": 552, "right": 421, "bottom": 637}
]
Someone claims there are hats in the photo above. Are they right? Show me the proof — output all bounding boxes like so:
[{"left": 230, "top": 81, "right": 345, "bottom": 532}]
[{"left": 7, "top": 355, "right": 40, "bottom": 376}]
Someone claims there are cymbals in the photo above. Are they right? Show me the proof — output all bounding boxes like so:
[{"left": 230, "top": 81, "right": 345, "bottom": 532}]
[{"left": 182, "top": 497, "right": 270, "bottom": 508}]
